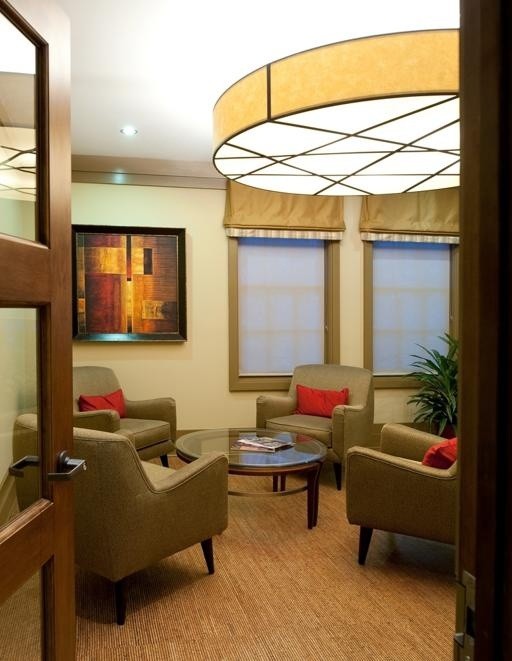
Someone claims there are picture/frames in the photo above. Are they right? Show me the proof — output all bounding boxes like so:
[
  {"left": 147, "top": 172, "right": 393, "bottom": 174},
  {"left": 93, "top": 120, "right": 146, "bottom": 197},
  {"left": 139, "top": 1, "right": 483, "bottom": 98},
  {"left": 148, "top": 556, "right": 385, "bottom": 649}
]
[{"left": 72, "top": 224, "right": 188, "bottom": 343}]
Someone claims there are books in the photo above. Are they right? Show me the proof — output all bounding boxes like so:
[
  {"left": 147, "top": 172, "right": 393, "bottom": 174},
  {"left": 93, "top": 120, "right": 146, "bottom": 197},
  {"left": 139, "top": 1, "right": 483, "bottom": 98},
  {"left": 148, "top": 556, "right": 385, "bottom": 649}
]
[{"left": 230, "top": 433, "right": 296, "bottom": 453}]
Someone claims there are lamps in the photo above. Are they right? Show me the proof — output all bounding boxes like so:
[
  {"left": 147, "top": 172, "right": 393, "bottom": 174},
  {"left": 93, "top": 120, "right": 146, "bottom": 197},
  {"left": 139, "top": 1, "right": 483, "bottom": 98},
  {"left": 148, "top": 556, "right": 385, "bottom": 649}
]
[
  {"left": 0, "top": 126, "right": 38, "bottom": 203},
  {"left": 210, "top": 28, "right": 460, "bottom": 197}
]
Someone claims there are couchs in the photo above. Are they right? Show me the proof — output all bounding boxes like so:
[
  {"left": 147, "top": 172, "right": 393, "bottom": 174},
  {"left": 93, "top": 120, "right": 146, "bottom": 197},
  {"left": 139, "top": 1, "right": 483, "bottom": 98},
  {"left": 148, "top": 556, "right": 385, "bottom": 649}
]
[
  {"left": 344, "top": 422, "right": 459, "bottom": 567},
  {"left": 12, "top": 414, "right": 230, "bottom": 626},
  {"left": 255, "top": 363, "right": 375, "bottom": 489},
  {"left": 72, "top": 367, "right": 178, "bottom": 469}
]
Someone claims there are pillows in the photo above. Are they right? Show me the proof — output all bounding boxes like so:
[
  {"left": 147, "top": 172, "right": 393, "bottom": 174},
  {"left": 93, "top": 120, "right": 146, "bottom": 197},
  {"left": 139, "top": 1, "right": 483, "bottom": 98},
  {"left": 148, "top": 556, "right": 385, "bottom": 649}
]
[
  {"left": 421, "top": 437, "right": 459, "bottom": 469},
  {"left": 291, "top": 384, "right": 350, "bottom": 418},
  {"left": 76, "top": 388, "right": 126, "bottom": 420}
]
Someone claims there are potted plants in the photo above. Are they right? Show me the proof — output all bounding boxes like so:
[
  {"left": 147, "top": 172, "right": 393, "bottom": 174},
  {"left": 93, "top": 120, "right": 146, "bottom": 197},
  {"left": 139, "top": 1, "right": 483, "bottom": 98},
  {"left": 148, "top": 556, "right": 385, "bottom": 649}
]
[{"left": 400, "top": 332, "right": 459, "bottom": 438}]
